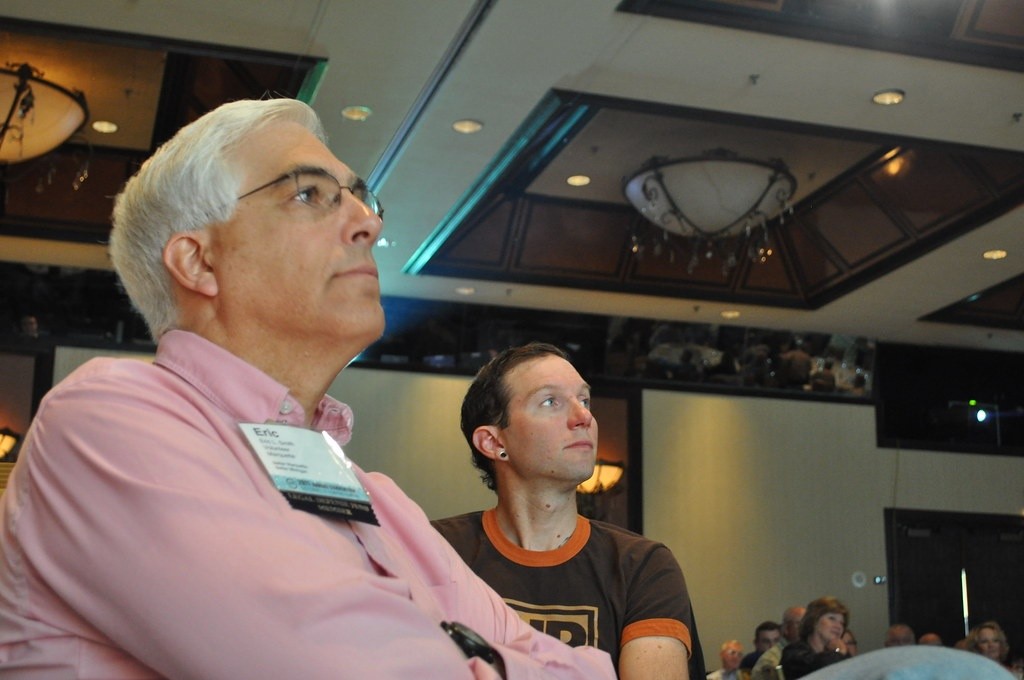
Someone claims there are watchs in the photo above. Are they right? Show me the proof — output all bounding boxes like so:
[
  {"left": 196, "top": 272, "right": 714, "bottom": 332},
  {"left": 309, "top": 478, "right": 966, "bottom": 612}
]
[{"left": 442, "top": 621, "right": 507, "bottom": 680}]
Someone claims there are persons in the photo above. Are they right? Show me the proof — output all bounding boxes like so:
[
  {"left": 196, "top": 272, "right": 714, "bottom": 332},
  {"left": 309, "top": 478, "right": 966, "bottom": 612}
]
[
  {"left": 706, "top": 640, "right": 744, "bottom": 680},
  {"left": 430, "top": 344, "right": 693, "bottom": 680},
  {"left": 779, "top": 598, "right": 852, "bottom": 680},
  {"left": 741, "top": 621, "right": 781, "bottom": 680},
  {"left": 885, "top": 625, "right": 915, "bottom": 647},
  {"left": 842, "top": 630, "right": 857, "bottom": 656},
  {"left": 0, "top": 99, "right": 617, "bottom": 680},
  {"left": 921, "top": 634, "right": 942, "bottom": 647},
  {"left": 956, "top": 624, "right": 1024, "bottom": 680},
  {"left": 751, "top": 606, "right": 805, "bottom": 680}
]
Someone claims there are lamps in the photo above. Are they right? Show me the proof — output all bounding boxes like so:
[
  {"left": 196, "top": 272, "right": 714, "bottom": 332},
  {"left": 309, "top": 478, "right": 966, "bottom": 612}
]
[
  {"left": 0, "top": 428, "right": 21, "bottom": 458},
  {"left": 622, "top": 149, "right": 797, "bottom": 265},
  {"left": 0, "top": 62, "right": 95, "bottom": 193},
  {"left": 977, "top": 409, "right": 994, "bottom": 423},
  {"left": 575, "top": 457, "right": 628, "bottom": 495}
]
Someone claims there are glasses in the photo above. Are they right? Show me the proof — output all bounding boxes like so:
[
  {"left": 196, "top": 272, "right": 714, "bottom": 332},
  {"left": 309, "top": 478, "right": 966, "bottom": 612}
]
[
  {"left": 723, "top": 649, "right": 743, "bottom": 658},
  {"left": 236, "top": 166, "right": 385, "bottom": 224}
]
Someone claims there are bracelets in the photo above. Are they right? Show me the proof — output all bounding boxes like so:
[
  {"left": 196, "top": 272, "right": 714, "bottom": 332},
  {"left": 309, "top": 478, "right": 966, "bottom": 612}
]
[{"left": 834, "top": 648, "right": 843, "bottom": 655}]
